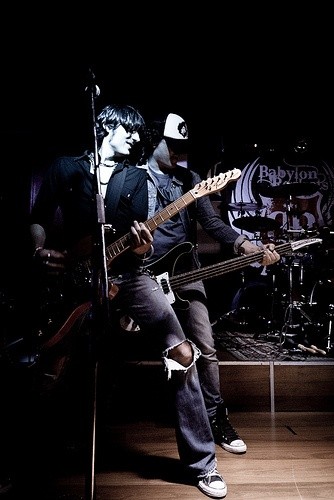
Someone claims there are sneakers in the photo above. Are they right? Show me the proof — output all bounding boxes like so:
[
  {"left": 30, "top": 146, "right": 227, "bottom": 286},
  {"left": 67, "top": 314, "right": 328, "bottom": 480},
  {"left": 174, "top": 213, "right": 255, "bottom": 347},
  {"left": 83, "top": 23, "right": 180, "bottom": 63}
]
[
  {"left": 196, "top": 469, "right": 227, "bottom": 497},
  {"left": 211, "top": 403, "right": 247, "bottom": 453}
]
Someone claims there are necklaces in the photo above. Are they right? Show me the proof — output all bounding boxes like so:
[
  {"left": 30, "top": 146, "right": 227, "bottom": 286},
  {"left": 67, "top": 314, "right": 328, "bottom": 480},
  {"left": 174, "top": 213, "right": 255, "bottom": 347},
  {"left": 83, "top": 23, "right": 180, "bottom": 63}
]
[
  {"left": 99, "top": 161, "right": 118, "bottom": 168},
  {"left": 100, "top": 181, "right": 109, "bottom": 185}
]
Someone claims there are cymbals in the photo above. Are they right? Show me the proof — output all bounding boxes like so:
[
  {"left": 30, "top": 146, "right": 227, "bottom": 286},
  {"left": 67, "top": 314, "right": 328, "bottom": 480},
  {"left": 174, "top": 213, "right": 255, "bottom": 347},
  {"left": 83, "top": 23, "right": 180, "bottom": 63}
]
[
  {"left": 217, "top": 202, "right": 268, "bottom": 212},
  {"left": 231, "top": 216, "right": 282, "bottom": 232},
  {"left": 258, "top": 182, "right": 321, "bottom": 198}
]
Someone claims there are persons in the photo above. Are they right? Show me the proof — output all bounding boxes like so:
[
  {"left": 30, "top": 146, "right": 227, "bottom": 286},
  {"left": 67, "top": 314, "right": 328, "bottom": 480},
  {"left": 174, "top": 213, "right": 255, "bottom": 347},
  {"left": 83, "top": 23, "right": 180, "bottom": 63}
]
[{"left": 23, "top": 106, "right": 282, "bottom": 497}]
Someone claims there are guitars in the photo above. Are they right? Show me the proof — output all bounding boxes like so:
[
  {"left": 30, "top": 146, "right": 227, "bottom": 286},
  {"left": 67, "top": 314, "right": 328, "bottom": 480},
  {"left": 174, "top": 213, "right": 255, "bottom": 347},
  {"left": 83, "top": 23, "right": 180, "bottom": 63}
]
[
  {"left": 0, "top": 166, "right": 243, "bottom": 378},
  {"left": 134, "top": 236, "right": 325, "bottom": 315}
]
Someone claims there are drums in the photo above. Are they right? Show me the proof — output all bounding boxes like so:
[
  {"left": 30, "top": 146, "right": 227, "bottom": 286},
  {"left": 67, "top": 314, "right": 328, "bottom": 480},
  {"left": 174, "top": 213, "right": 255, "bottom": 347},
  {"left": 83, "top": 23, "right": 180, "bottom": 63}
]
[{"left": 268, "top": 229, "right": 317, "bottom": 265}]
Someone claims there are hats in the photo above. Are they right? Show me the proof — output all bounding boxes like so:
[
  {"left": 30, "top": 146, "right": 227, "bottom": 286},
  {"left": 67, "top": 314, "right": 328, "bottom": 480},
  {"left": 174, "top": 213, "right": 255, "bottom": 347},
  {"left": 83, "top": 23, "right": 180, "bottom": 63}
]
[{"left": 149, "top": 111, "right": 192, "bottom": 141}]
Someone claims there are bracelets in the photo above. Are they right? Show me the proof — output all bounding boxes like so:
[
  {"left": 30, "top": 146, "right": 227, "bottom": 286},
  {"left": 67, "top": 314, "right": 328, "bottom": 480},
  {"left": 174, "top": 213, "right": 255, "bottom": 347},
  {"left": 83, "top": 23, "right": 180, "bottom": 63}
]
[{"left": 33, "top": 246, "right": 44, "bottom": 257}]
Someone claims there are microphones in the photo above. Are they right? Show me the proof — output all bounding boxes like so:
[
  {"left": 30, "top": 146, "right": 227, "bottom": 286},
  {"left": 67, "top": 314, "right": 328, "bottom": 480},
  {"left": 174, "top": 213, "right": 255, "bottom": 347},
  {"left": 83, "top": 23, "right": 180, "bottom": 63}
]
[{"left": 85, "top": 71, "right": 100, "bottom": 98}]
